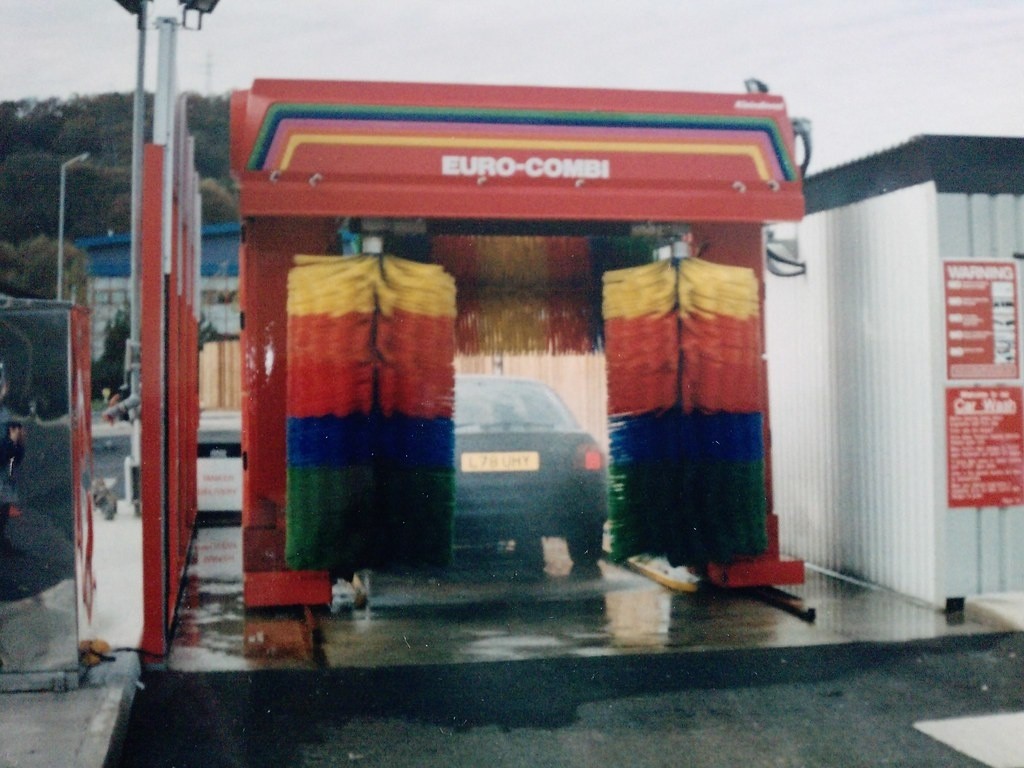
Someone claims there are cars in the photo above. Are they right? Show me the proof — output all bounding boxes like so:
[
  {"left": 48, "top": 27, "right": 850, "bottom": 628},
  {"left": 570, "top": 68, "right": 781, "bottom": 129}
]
[{"left": 447, "top": 370, "right": 615, "bottom": 570}]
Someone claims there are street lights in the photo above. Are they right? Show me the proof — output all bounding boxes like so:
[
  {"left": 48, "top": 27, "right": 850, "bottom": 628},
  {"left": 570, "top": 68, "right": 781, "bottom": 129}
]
[{"left": 51, "top": 152, "right": 103, "bottom": 300}]
